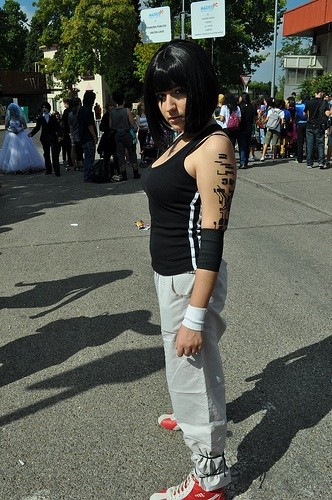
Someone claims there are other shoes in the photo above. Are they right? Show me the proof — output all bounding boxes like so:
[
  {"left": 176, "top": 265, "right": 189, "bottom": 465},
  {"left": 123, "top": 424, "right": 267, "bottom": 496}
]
[
  {"left": 133, "top": 169, "right": 140, "bottom": 179},
  {"left": 63, "top": 161, "right": 67, "bottom": 166},
  {"left": 318, "top": 164, "right": 323, "bottom": 167},
  {"left": 308, "top": 165, "right": 313, "bottom": 169},
  {"left": 239, "top": 164, "right": 247, "bottom": 169},
  {"left": 121, "top": 175, "right": 127, "bottom": 181},
  {"left": 252, "top": 157, "right": 260, "bottom": 161},
  {"left": 258, "top": 146, "right": 305, "bottom": 163}
]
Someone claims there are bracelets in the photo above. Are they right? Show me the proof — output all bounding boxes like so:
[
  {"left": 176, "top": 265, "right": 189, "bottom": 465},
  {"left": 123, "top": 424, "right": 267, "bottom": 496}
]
[{"left": 181, "top": 304, "right": 207, "bottom": 332}]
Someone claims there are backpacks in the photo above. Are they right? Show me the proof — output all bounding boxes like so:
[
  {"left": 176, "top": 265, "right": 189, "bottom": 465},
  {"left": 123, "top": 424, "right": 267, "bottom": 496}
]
[
  {"left": 266, "top": 108, "right": 282, "bottom": 129},
  {"left": 257, "top": 107, "right": 270, "bottom": 128},
  {"left": 227, "top": 105, "right": 239, "bottom": 131}
]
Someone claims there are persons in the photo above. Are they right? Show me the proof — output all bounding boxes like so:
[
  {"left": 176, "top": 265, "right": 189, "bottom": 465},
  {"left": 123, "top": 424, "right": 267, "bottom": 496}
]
[
  {"left": 141, "top": 40, "right": 237, "bottom": 500},
  {"left": 4, "top": 103, "right": 28, "bottom": 174},
  {"left": 108, "top": 90, "right": 142, "bottom": 181},
  {"left": 27, "top": 101, "right": 63, "bottom": 177},
  {"left": 76, "top": 89, "right": 99, "bottom": 182},
  {"left": 52, "top": 88, "right": 332, "bottom": 170}
]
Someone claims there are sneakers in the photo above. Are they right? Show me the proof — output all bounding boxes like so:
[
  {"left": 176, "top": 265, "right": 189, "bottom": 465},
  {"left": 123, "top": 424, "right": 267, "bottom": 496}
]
[
  {"left": 148, "top": 469, "right": 226, "bottom": 500},
  {"left": 159, "top": 413, "right": 181, "bottom": 431}
]
